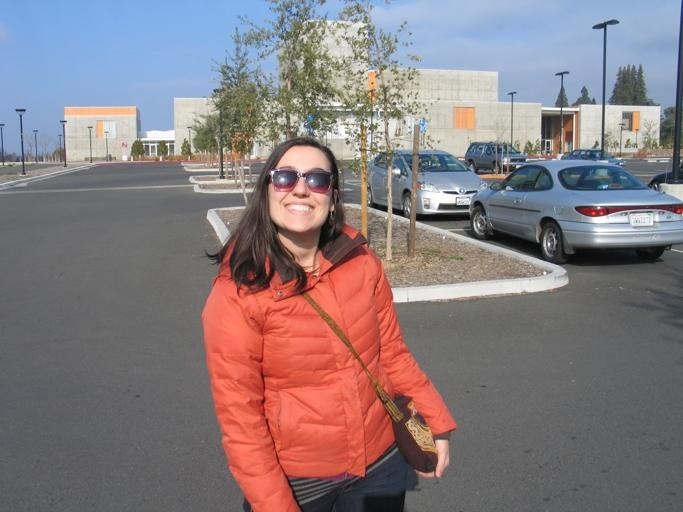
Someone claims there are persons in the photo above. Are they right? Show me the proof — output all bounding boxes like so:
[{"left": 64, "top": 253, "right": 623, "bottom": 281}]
[{"left": 201, "top": 136, "right": 457, "bottom": 512}]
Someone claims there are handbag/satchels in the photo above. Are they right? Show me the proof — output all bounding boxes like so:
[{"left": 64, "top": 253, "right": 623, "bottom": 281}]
[{"left": 387, "top": 393, "right": 439, "bottom": 473}]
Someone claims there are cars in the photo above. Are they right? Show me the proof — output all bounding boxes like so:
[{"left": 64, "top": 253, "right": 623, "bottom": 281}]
[
  {"left": 466, "top": 158, "right": 682, "bottom": 265},
  {"left": 365, "top": 146, "right": 488, "bottom": 222},
  {"left": 560, "top": 146, "right": 624, "bottom": 168},
  {"left": 645, "top": 165, "right": 682, "bottom": 193}
]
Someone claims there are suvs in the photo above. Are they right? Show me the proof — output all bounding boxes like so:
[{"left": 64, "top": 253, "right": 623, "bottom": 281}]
[{"left": 460, "top": 140, "right": 528, "bottom": 172}]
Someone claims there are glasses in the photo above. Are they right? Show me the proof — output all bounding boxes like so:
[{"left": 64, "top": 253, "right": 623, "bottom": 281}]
[{"left": 268, "top": 169, "right": 336, "bottom": 194}]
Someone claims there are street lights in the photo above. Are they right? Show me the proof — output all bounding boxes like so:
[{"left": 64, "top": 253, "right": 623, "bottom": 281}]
[
  {"left": 591, "top": 18, "right": 621, "bottom": 160},
  {"left": 0, "top": 123, "right": 5, "bottom": 167},
  {"left": 14, "top": 107, "right": 27, "bottom": 175},
  {"left": 186, "top": 126, "right": 194, "bottom": 160},
  {"left": 57, "top": 134, "right": 63, "bottom": 162},
  {"left": 59, "top": 119, "right": 69, "bottom": 167},
  {"left": 634, "top": 128, "right": 639, "bottom": 148},
  {"left": 553, "top": 70, "right": 570, "bottom": 154},
  {"left": 211, "top": 86, "right": 226, "bottom": 179},
  {"left": 32, "top": 129, "right": 39, "bottom": 162},
  {"left": 507, "top": 90, "right": 516, "bottom": 145},
  {"left": 103, "top": 130, "right": 110, "bottom": 161},
  {"left": 617, "top": 123, "right": 625, "bottom": 157},
  {"left": 87, "top": 126, "right": 94, "bottom": 163}
]
